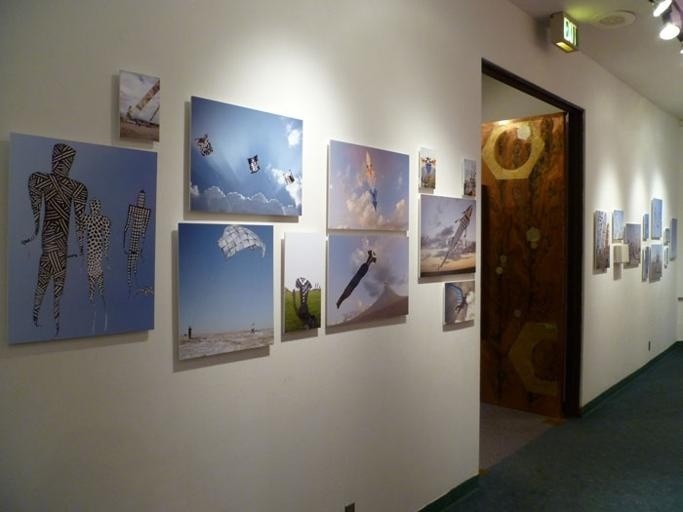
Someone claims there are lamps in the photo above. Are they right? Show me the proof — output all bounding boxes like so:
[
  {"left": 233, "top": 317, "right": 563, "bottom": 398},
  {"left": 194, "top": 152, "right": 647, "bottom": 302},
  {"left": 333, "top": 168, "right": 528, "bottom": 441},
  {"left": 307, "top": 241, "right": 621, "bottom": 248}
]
[{"left": 648, "top": 0, "right": 683, "bottom": 44}]
